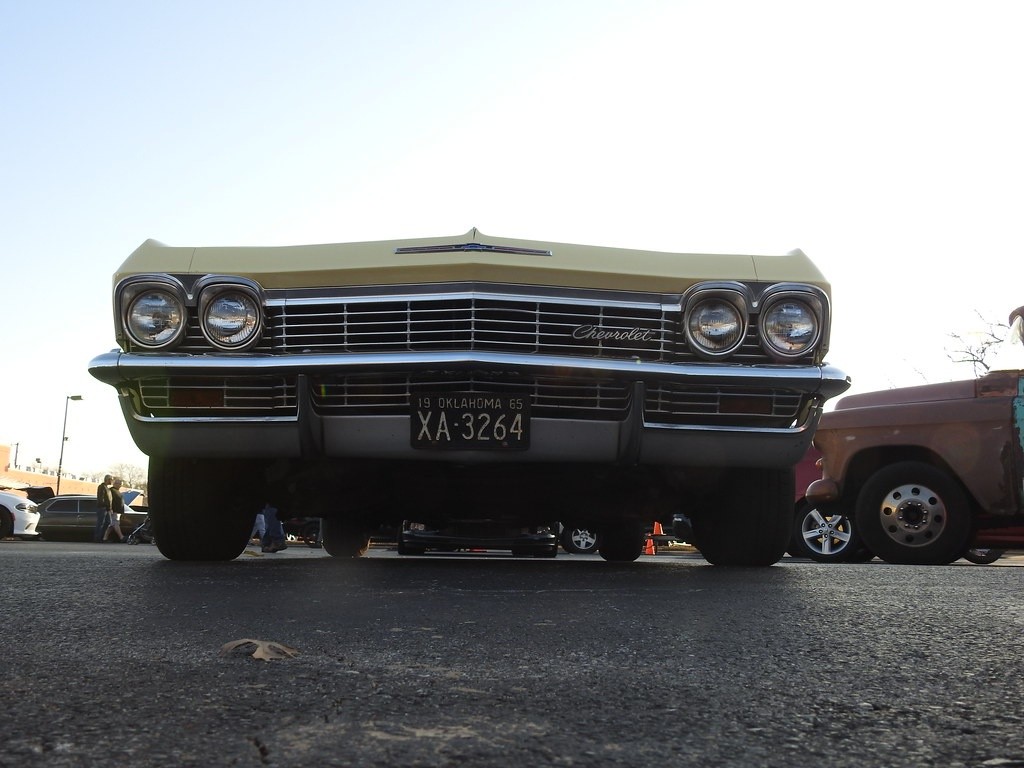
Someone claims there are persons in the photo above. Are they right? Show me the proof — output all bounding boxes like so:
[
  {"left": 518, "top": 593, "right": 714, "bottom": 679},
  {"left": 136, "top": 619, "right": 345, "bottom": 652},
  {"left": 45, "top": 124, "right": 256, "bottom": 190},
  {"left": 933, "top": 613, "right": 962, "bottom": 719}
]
[
  {"left": 103, "top": 477, "right": 128, "bottom": 542},
  {"left": 95, "top": 475, "right": 113, "bottom": 543},
  {"left": 262, "top": 513, "right": 288, "bottom": 552},
  {"left": 248, "top": 512, "right": 265, "bottom": 546}
]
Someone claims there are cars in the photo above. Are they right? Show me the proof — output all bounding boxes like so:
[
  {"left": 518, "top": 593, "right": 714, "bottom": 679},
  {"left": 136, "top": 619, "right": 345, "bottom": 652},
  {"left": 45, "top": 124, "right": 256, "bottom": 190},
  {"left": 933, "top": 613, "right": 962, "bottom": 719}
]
[
  {"left": 87, "top": 226, "right": 852, "bottom": 567},
  {"left": 0, "top": 491, "right": 43, "bottom": 541},
  {"left": 280, "top": 513, "right": 400, "bottom": 549},
  {"left": 35, "top": 489, "right": 149, "bottom": 541},
  {"left": 786, "top": 440, "right": 1024, "bottom": 564},
  {"left": 399, "top": 516, "right": 560, "bottom": 560},
  {"left": 811, "top": 305, "right": 1024, "bottom": 564}
]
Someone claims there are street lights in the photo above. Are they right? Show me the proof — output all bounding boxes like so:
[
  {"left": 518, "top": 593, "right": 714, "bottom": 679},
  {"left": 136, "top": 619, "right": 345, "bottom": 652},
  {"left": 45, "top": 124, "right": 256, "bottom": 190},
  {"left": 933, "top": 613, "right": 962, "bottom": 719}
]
[{"left": 56, "top": 395, "right": 84, "bottom": 495}]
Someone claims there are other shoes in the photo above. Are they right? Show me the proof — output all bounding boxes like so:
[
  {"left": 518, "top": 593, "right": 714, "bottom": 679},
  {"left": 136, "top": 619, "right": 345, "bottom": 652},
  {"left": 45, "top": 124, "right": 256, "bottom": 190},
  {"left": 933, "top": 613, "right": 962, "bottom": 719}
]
[
  {"left": 118, "top": 536, "right": 128, "bottom": 543},
  {"left": 102, "top": 538, "right": 114, "bottom": 543}
]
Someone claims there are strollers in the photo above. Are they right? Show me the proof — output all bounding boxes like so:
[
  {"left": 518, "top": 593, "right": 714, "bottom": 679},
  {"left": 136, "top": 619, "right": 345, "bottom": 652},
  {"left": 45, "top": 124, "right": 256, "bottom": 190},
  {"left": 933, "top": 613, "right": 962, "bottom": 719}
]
[{"left": 123, "top": 514, "right": 157, "bottom": 546}]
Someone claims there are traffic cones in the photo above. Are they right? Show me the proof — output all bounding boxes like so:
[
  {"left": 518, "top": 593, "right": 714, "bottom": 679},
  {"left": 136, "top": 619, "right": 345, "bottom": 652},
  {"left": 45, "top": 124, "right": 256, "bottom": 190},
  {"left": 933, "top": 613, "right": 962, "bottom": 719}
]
[
  {"left": 648, "top": 521, "right": 667, "bottom": 537},
  {"left": 644, "top": 533, "right": 656, "bottom": 555}
]
